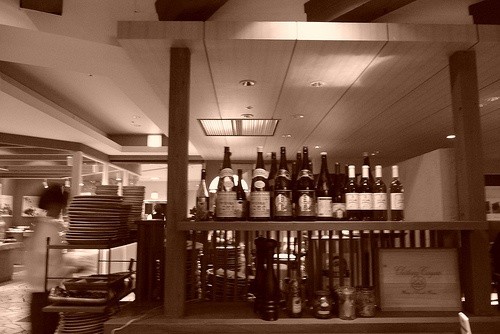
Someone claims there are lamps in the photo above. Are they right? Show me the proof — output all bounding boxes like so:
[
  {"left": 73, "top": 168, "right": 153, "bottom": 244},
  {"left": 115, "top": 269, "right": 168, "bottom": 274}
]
[
  {"left": 67, "top": 157, "right": 122, "bottom": 180},
  {"left": 42, "top": 178, "right": 48, "bottom": 189},
  {"left": 65, "top": 178, "right": 71, "bottom": 188}
]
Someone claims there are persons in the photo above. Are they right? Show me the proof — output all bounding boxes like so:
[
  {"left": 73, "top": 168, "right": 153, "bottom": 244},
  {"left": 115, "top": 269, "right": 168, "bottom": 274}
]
[{"left": 30, "top": 181, "right": 89, "bottom": 334}]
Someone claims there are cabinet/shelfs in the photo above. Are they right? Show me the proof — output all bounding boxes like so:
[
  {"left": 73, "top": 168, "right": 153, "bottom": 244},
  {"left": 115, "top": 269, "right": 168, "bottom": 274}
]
[
  {"left": 103, "top": 221, "right": 499, "bottom": 334},
  {"left": 44, "top": 236, "right": 138, "bottom": 320}
]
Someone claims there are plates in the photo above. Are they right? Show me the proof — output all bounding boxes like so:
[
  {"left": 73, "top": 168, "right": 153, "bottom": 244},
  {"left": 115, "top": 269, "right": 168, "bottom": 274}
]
[
  {"left": 57, "top": 183, "right": 144, "bottom": 334},
  {"left": 198, "top": 245, "right": 251, "bottom": 301}
]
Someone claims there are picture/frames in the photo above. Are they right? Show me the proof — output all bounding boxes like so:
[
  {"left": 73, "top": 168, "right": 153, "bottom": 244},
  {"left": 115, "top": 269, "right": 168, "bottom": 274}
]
[{"left": 377, "top": 249, "right": 462, "bottom": 311}]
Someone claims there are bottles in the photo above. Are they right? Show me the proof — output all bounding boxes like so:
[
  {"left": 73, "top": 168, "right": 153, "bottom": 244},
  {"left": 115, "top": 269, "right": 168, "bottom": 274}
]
[
  {"left": 256, "top": 237, "right": 374, "bottom": 320},
  {"left": 216, "top": 146, "right": 387, "bottom": 220},
  {"left": 389, "top": 165, "right": 404, "bottom": 221},
  {"left": 196, "top": 169, "right": 210, "bottom": 221}
]
